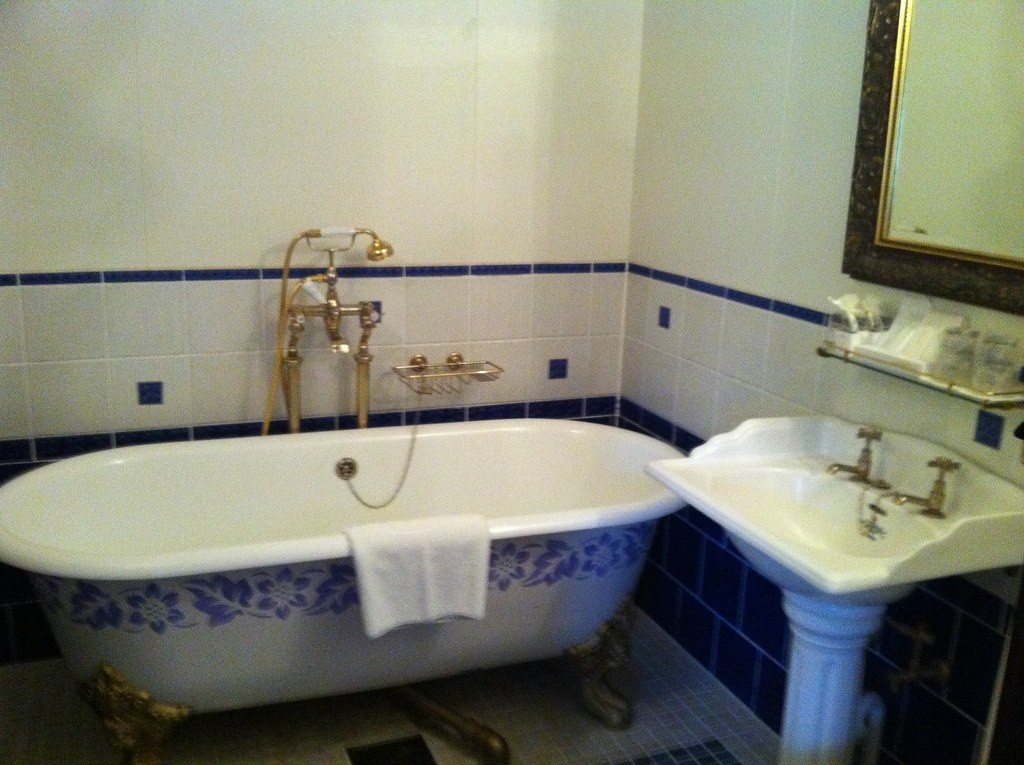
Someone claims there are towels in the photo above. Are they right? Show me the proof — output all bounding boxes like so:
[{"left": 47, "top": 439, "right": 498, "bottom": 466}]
[{"left": 343, "top": 514, "right": 491, "bottom": 639}]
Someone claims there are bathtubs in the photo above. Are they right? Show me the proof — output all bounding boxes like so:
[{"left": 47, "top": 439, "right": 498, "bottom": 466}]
[{"left": 0, "top": 409, "right": 717, "bottom": 756}]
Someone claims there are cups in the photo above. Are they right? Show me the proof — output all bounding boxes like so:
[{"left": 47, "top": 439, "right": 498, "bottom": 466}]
[
  {"left": 937, "top": 328, "right": 981, "bottom": 386},
  {"left": 971, "top": 335, "right": 1024, "bottom": 393}
]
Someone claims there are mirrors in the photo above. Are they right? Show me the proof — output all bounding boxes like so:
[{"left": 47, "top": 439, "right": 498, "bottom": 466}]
[{"left": 837, "top": 0, "right": 1024, "bottom": 320}]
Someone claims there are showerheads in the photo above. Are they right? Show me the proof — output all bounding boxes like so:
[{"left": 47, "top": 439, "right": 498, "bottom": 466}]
[{"left": 308, "top": 227, "right": 394, "bottom": 262}]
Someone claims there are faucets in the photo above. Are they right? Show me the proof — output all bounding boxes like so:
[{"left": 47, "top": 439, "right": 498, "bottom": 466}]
[
  {"left": 827, "top": 429, "right": 893, "bottom": 489},
  {"left": 896, "top": 456, "right": 962, "bottom": 517}
]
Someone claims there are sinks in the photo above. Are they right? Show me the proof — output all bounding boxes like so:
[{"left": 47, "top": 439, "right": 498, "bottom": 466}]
[{"left": 643, "top": 414, "right": 1024, "bottom": 596}]
